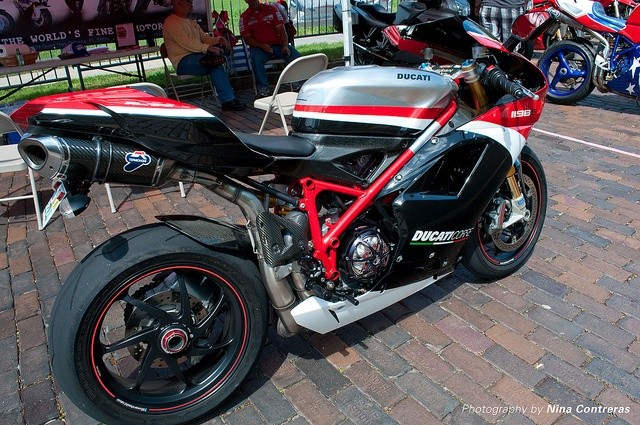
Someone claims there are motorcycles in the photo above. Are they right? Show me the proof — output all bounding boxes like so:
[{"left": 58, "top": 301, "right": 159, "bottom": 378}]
[
  {"left": 334, "top": 0, "right": 470, "bottom": 65},
  {"left": 537, "top": 0, "right": 640, "bottom": 102},
  {"left": 10, "top": 3, "right": 548, "bottom": 423},
  {"left": 501, "top": 0, "right": 577, "bottom": 61}
]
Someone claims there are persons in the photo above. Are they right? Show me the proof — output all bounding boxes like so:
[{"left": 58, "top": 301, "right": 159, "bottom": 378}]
[
  {"left": 474, "top": 0, "right": 524, "bottom": 52},
  {"left": 276, "top": 0, "right": 296, "bottom": 47},
  {"left": 240, "top": 0, "right": 303, "bottom": 96},
  {"left": 162, "top": 0, "right": 246, "bottom": 111}
]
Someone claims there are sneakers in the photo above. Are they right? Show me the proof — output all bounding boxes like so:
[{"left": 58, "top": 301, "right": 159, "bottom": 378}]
[
  {"left": 200, "top": 55, "right": 226, "bottom": 66},
  {"left": 258, "top": 87, "right": 270, "bottom": 96},
  {"left": 221, "top": 99, "right": 246, "bottom": 111}
]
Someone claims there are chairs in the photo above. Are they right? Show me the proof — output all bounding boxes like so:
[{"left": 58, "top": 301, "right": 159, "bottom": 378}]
[
  {"left": 103, "top": 82, "right": 187, "bottom": 214},
  {"left": 242, "top": 34, "right": 293, "bottom": 95},
  {"left": 253, "top": 53, "right": 329, "bottom": 136},
  {"left": 160, "top": 40, "right": 222, "bottom": 108},
  {"left": 0, "top": 111, "right": 43, "bottom": 231}
]
